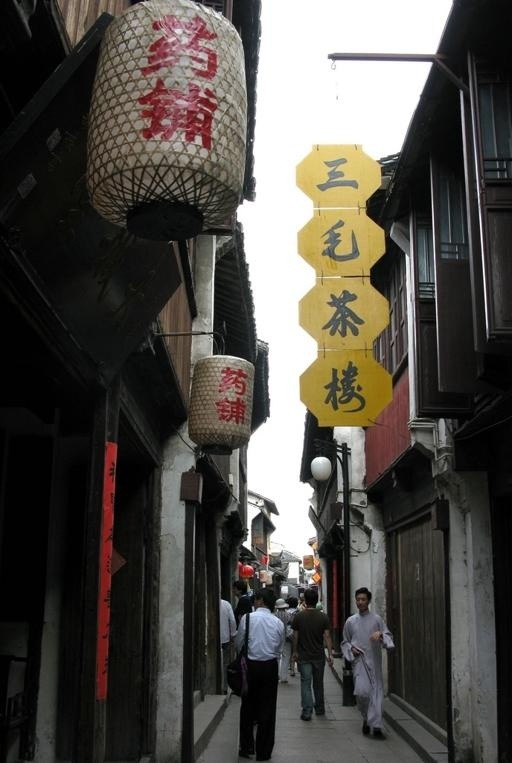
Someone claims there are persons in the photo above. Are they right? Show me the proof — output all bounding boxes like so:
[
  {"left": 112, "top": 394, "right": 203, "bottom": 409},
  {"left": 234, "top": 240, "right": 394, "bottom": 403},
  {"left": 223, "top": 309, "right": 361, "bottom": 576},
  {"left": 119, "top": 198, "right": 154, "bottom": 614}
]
[
  {"left": 340, "top": 584, "right": 396, "bottom": 740},
  {"left": 220, "top": 578, "right": 334, "bottom": 720},
  {"left": 234, "top": 586, "right": 288, "bottom": 762}
]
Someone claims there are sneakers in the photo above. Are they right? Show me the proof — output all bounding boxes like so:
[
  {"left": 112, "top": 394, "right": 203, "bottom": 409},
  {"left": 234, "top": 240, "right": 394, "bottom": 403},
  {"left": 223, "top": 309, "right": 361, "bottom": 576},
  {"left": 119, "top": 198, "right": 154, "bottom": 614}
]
[
  {"left": 362, "top": 718, "right": 371, "bottom": 735},
  {"left": 373, "top": 727, "right": 386, "bottom": 740},
  {"left": 239, "top": 747, "right": 255, "bottom": 755}
]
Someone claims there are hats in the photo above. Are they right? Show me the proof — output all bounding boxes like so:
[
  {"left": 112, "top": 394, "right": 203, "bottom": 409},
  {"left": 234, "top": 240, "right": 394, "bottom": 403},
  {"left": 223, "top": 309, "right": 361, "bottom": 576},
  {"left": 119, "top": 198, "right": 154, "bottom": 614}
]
[{"left": 274, "top": 598, "right": 290, "bottom": 609}]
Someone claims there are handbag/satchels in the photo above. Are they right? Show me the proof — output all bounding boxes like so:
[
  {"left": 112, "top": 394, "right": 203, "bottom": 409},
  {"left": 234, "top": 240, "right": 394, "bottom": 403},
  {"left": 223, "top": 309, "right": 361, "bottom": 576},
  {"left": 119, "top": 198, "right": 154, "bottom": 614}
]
[{"left": 226, "top": 656, "right": 243, "bottom": 696}]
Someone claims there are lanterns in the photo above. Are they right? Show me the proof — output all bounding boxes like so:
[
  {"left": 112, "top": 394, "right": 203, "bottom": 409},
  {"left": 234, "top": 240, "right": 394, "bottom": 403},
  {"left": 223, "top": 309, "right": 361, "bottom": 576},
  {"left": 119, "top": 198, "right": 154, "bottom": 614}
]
[
  {"left": 86, "top": 0, "right": 248, "bottom": 241},
  {"left": 187, "top": 355, "right": 255, "bottom": 453}
]
